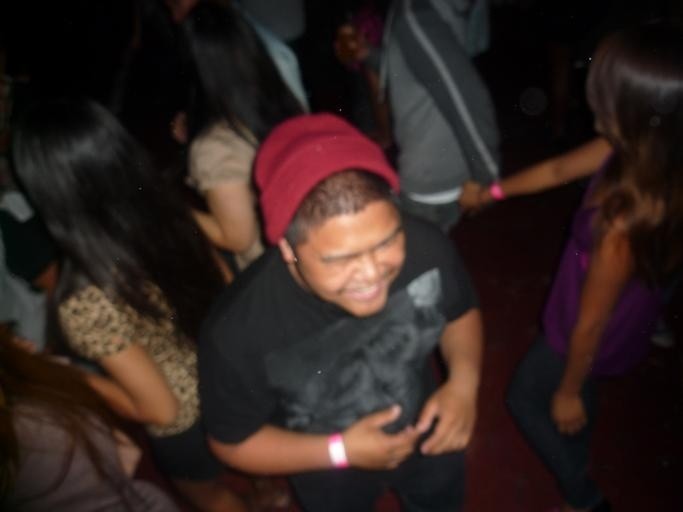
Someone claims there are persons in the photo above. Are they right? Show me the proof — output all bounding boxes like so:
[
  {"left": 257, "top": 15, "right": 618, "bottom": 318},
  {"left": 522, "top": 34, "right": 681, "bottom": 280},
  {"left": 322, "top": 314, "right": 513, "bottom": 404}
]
[
  {"left": 336, "top": 1, "right": 680, "bottom": 511},
  {"left": 2, "top": 1, "right": 335, "bottom": 512},
  {"left": 196, "top": 114, "right": 484, "bottom": 511}
]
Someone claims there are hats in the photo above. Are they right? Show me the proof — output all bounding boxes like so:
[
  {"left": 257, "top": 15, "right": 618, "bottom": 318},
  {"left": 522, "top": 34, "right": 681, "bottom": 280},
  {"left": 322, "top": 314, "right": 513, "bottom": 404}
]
[{"left": 254, "top": 113, "right": 401, "bottom": 246}]
